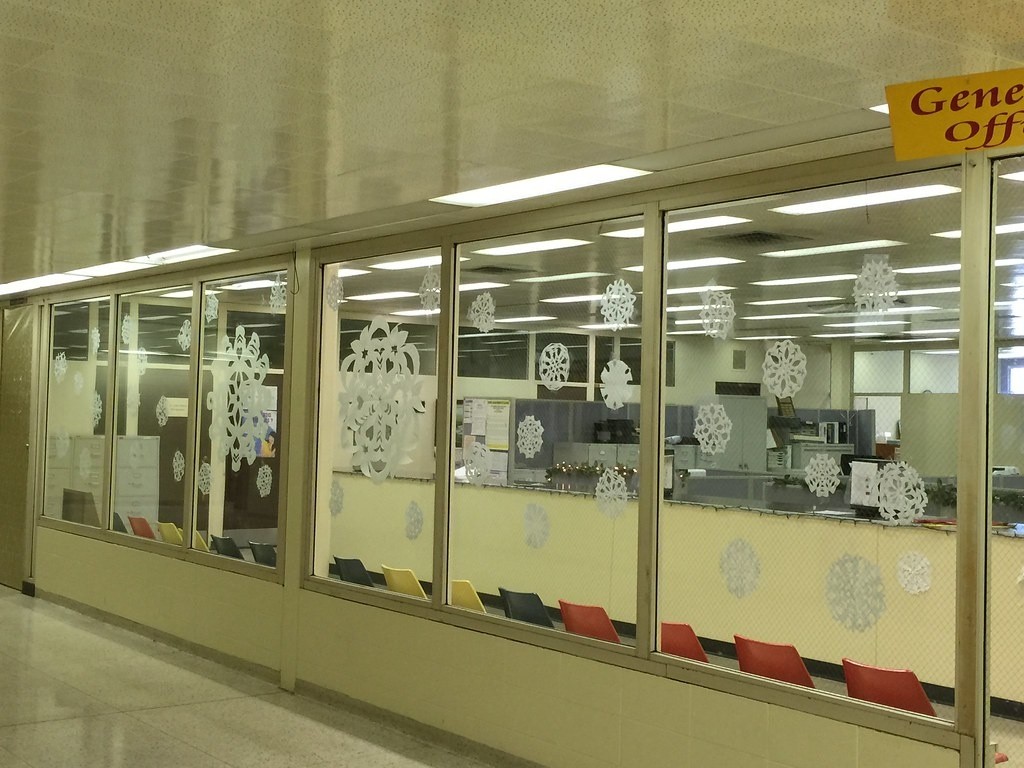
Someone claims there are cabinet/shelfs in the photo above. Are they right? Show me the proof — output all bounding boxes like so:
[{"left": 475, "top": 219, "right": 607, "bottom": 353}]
[
  {"left": 766, "top": 445, "right": 792, "bottom": 471},
  {"left": 46, "top": 433, "right": 160, "bottom": 537}
]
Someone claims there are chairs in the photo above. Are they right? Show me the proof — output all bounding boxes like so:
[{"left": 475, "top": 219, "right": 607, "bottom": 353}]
[
  {"left": 333, "top": 555, "right": 375, "bottom": 587},
  {"left": 558, "top": 599, "right": 620, "bottom": 643},
  {"left": 842, "top": 658, "right": 1009, "bottom": 765},
  {"left": 498, "top": 586, "right": 555, "bottom": 628},
  {"left": 733, "top": 633, "right": 816, "bottom": 689},
  {"left": 380, "top": 564, "right": 427, "bottom": 598},
  {"left": 452, "top": 580, "right": 486, "bottom": 612},
  {"left": 127, "top": 516, "right": 156, "bottom": 539},
  {"left": 113, "top": 512, "right": 128, "bottom": 533},
  {"left": 210, "top": 534, "right": 245, "bottom": 560},
  {"left": 248, "top": 540, "right": 277, "bottom": 567},
  {"left": 156, "top": 521, "right": 183, "bottom": 545},
  {"left": 661, "top": 622, "right": 709, "bottom": 663},
  {"left": 177, "top": 528, "right": 210, "bottom": 553}
]
